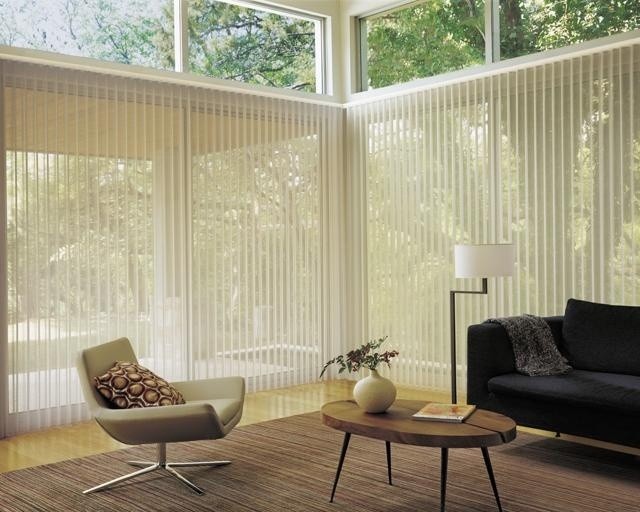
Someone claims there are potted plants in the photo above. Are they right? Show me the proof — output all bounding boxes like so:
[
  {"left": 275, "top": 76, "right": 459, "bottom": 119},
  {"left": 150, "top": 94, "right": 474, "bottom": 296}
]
[{"left": 319, "top": 337, "right": 399, "bottom": 414}]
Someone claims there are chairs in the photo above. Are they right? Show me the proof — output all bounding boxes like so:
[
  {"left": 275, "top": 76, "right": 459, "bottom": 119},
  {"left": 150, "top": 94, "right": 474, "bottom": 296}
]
[{"left": 75, "top": 337, "right": 247, "bottom": 496}]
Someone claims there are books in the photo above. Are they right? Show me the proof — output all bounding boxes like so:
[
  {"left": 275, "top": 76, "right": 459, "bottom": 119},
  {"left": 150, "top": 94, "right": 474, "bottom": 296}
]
[{"left": 412, "top": 402, "right": 478, "bottom": 423}]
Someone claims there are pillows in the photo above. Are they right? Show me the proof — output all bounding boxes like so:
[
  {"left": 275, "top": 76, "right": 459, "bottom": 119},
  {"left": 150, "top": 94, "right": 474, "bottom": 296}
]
[
  {"left": 94, "top": 361, "right": 185, "bottom": 408},
  {"left": 562, "top": 298, "right": 640, "bottom": 377}
]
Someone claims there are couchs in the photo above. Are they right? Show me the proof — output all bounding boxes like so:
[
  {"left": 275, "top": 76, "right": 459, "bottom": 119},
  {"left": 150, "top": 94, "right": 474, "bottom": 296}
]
[{"left": 466, "top": 315, "right": 640, "bottom": 448}]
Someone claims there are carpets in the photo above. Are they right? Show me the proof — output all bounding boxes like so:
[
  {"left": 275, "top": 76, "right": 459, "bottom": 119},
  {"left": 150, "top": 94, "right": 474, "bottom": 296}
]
[{"left": 0, "top": 410, "right": 640, "bottom": 512}]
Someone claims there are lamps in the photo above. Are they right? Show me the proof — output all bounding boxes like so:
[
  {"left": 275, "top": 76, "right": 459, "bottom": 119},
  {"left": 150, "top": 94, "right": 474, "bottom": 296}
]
[{"left": 450, "top": 243, "right": 515, "bottom": 404}]
